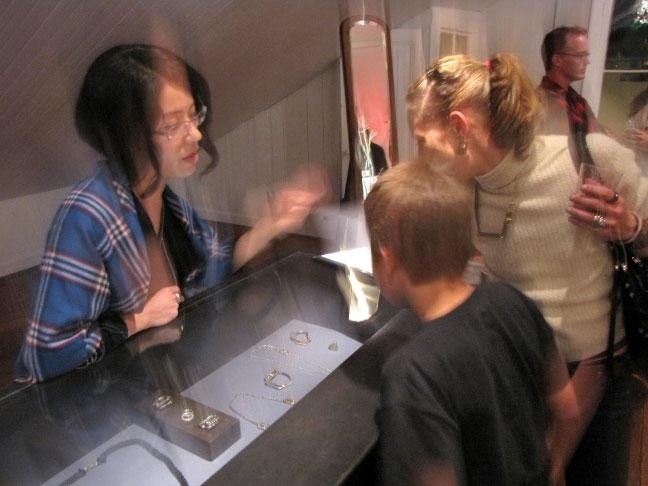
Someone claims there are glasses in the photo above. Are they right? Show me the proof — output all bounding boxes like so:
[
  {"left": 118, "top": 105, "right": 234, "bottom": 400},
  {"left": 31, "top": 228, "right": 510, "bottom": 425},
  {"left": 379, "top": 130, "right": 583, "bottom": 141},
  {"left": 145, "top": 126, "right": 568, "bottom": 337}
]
[
  {"left": 562, "top": 51, "right": 590, "bottom": 60},
  {"left": 152, "top": 105, "right": 208, "bottom": 140}
]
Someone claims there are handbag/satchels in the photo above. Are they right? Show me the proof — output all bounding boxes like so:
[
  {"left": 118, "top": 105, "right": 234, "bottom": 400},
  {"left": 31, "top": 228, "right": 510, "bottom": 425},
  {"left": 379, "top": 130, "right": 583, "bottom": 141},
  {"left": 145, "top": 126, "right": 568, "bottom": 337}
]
[{"left": 566, "top": 131, "right": 648, "bottom": 383}]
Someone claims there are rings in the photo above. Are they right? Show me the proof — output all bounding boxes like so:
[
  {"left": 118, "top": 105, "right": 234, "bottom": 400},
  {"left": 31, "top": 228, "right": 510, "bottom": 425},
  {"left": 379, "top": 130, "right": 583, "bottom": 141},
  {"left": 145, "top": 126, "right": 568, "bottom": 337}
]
[
  {"left": 592, "top": 214, "right": 606, "bottom": 228},
  {"left": 175, "top": 293, "right": 181, "bottom": 302},
  {"left": 182, "top": 407, "right": 194, "bottom": 421},
  {"left": 608, "top": 192, "right": 619, "bottom": 204}
]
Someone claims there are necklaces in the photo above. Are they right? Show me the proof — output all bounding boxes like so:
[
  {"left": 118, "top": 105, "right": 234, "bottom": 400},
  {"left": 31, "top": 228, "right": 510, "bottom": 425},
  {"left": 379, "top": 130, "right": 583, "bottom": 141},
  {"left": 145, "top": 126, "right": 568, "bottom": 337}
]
[
  {"left": 466, "top": 180, "right": 518, "bottom": 241},
  {"left": 228, "top": 391, "right": 295, "bottom": 431},
  {"left": 250, "top": 343, "right": 333, "bottom": 377},
  {"left": 58, "top": 438, "right": 190, "bottom": 486}
]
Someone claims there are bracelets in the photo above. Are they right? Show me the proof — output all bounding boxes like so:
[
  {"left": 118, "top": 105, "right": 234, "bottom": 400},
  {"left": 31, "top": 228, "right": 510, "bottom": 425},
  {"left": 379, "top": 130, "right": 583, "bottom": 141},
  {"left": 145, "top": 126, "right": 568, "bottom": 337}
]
[
  {"left": 198, "top": 414, "right": 220, "bottom": 430},
  {"left": 263, "top": 369, "right": 294, "bottom": 391},
  {"left": 617, "top": 210, "right": 642, "bottom": 245},
  {"left": 288, "top": 329, "right": 312, "bottom": 346}
]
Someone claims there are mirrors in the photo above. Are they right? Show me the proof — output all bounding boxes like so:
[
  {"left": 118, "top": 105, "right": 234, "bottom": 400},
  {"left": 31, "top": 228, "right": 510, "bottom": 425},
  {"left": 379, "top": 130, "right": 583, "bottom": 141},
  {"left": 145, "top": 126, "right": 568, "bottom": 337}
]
[{"left": 340, "top": 14, "right": 399, "bottom": 203}]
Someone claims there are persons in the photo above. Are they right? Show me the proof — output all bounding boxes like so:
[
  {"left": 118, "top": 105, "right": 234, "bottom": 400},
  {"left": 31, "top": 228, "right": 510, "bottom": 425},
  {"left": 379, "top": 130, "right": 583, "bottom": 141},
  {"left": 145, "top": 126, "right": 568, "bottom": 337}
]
[
  {"left": 361, "top": 159, "right": 583, "bottom": 486},
  {"left": 622, "top": 106, "right": 647, "bottom": 175},
  {"left": 12, "top": 43, "right": 327, "bottom": 387},
  {"left": 406, "top": 50, "right": 648, "bottom": 485},
  {"left": 532, "top": 24, "right": 604, "bottom": 165}
]
